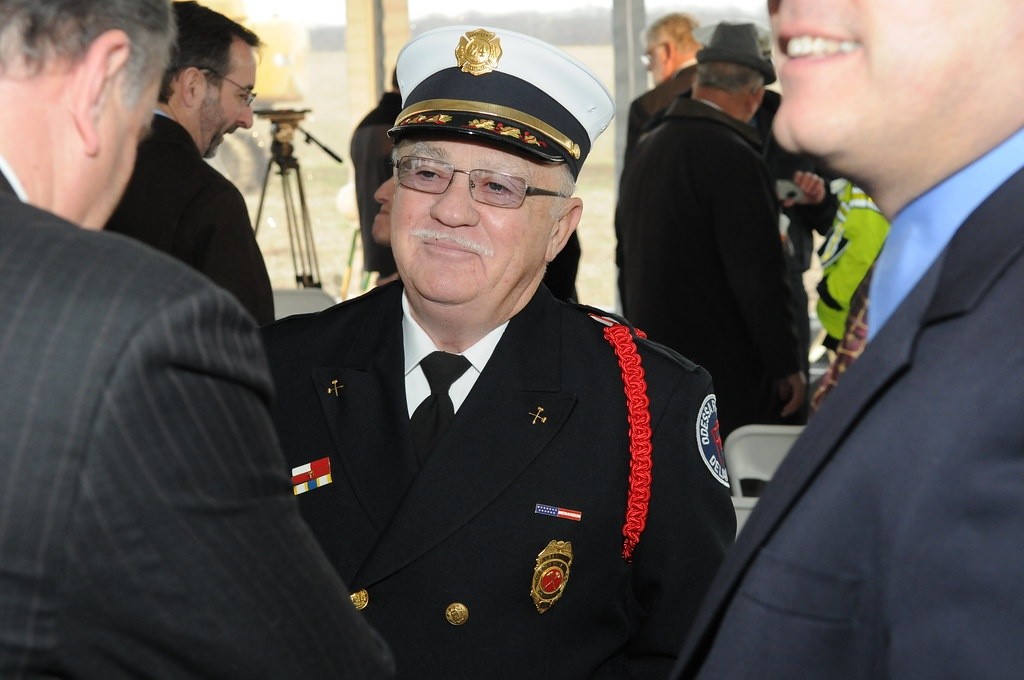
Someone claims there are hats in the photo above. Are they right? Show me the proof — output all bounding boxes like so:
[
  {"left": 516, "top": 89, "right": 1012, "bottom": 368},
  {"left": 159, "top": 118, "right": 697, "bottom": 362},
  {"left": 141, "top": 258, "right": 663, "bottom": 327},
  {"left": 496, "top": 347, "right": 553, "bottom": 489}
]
[
  {"left": 394, "top": 26, "right": 615, "bottom": 182},
  {"left": 696, "top": 22, "right": 776, "bottom": 85}
]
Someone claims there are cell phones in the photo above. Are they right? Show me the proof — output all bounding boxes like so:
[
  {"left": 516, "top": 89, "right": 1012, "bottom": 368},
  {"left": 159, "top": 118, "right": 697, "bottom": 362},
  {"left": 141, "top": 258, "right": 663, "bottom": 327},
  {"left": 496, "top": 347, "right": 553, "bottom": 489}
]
[{"left": 774, "top": 180, "right": 803, "bottom": 202}]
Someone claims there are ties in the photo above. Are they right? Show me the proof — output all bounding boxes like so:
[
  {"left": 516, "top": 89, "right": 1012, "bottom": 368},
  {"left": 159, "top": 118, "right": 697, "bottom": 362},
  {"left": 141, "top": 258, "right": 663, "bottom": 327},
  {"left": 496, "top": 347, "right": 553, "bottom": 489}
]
[
  {"left": 408, "top": 351, "right": 470, "bottom": 467},
  {"left": 804, "top": 259, "right": 872, "bottom": 408}
]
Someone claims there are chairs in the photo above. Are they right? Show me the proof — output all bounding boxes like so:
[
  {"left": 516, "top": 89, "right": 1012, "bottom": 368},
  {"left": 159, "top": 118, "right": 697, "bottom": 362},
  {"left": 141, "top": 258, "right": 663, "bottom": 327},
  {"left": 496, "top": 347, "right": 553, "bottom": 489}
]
[{"left": 724, "top": 425, "right": 808, "bottom": 542}]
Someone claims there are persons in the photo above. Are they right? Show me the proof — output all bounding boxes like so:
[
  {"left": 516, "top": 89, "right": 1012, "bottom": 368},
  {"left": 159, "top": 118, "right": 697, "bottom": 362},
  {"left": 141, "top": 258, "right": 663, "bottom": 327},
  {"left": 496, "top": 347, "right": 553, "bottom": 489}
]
[
  {"left": 258, "top": 23, "right": 738, "bottom": 680},
  {"left": 352, "top": 68, "right": 580, "bottom": 305},
  {"left": 0, "top": 0, "right": 398, "bottom": 680},
  {"left": 103, "top": 2, "right": 275, "bottom": 327},
  {"left": 613, "top": 11, "right": 892, "bottom": 496},
  {"left": 673, "top": 0, "right": 1023, "bottom": 679}
]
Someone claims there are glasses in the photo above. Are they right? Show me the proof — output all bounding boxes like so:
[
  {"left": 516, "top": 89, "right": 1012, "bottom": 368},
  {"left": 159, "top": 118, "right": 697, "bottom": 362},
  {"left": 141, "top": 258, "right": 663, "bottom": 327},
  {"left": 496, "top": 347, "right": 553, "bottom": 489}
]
[
  {"left": 173, "top": 64, "right": 258, "bottom": 111},
  {"left": 640, "top": 43, "right": 665, "bottom": 68},
  {"left": 394, "top": 153, "right": 571, "bottom": 210}
]
